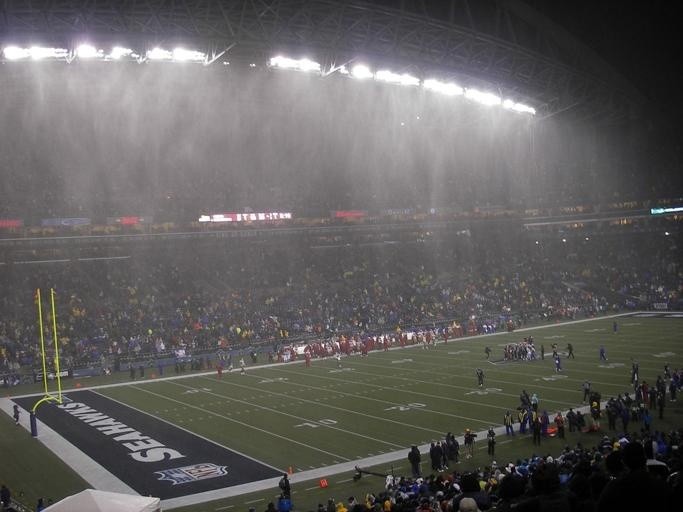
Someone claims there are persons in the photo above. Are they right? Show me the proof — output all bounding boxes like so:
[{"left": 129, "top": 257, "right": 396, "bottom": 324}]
[
  {"left": 283, "top": 368, "right": 683, "bottom": 510},
  {"left": 0, "top": 215, "right": 682, "bottom": 389},
  {"left": 0, "top": 483, "right": 10, "bottom": 507},
  {"left": 34, "top": 497, "right": 46, "bottom": 511},
  {"left": 12, "top": 404, "right": 21, "bottom": 426}
]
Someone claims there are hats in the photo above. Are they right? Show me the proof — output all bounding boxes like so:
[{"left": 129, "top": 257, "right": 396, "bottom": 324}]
[{"left": 458, "top": 427, "right": 647, "bottom": 512}]
